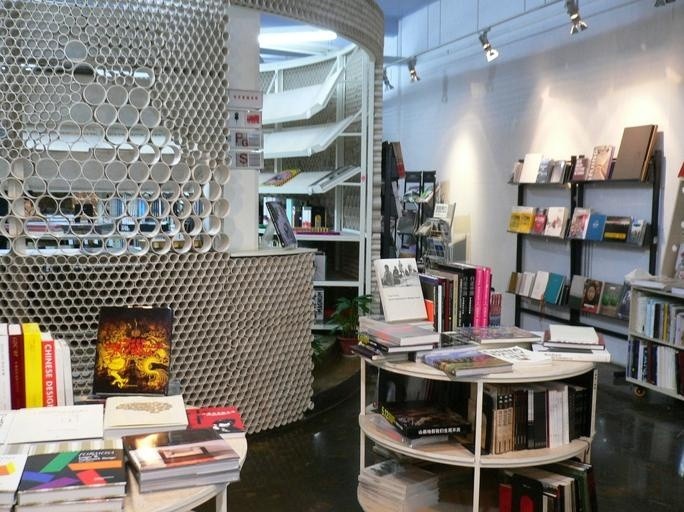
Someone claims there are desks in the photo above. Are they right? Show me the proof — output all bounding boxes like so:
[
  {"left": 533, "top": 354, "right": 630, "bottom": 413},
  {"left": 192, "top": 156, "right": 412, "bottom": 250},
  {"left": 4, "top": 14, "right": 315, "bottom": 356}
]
[{"left": 0, "top": 390, "right": 249, "bottom": 507}]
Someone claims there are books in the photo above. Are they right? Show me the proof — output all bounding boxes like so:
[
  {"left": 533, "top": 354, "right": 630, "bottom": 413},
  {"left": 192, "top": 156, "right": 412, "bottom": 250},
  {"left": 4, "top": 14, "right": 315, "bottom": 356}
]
[
  {"left": 25, "top": 196, "right": 208, "bottom": 252},
  {"left": 350, "top": 256, "right": 612, "bottom": 511},
  {"left": 262, "top": 168, "right": 301, "bottom": 187},
  {"left": 262, "top": 196, "right": 328, "bottom": 249},
  {"left": 507, "top": 124, "right": 659, "bottom": 323},
  {"left": 625, "top": 290, "right": 684, "bottom": 397},
  {"left": 0, "top": 307, "right": 246, "bottom": 512},
  {"left": 397, "top": 182, "right": 452, "bottom": 257}
]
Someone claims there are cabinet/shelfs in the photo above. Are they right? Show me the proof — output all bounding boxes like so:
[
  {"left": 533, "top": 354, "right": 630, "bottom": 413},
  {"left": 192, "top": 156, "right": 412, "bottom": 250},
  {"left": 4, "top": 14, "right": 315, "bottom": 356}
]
[
  {"left": 619, "top": 268, "right": 684, "bottom": 399},
  {"left": 354, "top": 334, "right": 606, "bottom": 512},
  {"left": 504, "top": 155, "right": 664, "bottom": 392},
  {"left": 256, "top": 49, "right": 368, "bottom": 333}
]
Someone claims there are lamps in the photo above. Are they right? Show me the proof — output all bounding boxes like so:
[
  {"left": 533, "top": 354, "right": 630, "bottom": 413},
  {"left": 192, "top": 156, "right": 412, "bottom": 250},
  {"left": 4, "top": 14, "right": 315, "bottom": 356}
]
[{"left": 565, "top": 1, "right": 588, "bottom": 36}]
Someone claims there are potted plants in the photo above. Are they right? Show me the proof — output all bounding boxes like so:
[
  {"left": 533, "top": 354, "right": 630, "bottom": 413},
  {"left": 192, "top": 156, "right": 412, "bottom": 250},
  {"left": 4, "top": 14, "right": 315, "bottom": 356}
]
[{"left": 328, "top": 292, "right": 374, "bottom": 358}]
